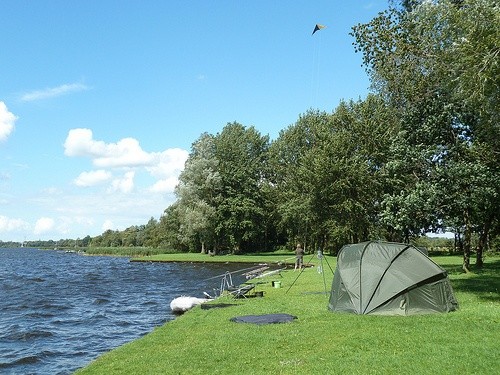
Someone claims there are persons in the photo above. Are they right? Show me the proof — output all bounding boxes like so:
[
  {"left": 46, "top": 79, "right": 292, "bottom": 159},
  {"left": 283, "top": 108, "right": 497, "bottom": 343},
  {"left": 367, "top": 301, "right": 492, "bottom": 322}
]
[{"left": 293, "top": 243, "right": 305, "bottom": 273}]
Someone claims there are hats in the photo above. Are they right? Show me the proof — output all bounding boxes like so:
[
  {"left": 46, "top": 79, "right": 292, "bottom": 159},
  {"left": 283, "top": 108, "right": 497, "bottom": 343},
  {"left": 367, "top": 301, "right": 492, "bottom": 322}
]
[{"left": 297, "top": 244, "right": 301, "bottom": 247}]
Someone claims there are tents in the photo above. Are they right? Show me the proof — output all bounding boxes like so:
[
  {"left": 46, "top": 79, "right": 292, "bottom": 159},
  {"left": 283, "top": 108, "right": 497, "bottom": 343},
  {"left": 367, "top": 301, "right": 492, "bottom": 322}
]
[{"left": 325, "top": 240, "right": 460, "bottom": 317}]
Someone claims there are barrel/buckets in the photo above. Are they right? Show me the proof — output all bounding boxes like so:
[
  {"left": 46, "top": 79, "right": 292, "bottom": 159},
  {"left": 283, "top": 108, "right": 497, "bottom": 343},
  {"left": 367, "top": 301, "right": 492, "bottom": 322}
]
[
  {"left": 274, "top": 282, "right": 281, "bottom": 288},
  {"left": 256, "top": 292, "right": 263, "bottom": 297}
]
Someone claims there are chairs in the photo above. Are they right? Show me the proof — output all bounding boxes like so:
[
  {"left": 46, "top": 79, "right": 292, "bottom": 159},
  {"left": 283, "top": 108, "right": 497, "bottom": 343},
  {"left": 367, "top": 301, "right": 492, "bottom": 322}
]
[{"left": 228, "top": 286, "right": 255, "bottom": 298}]
